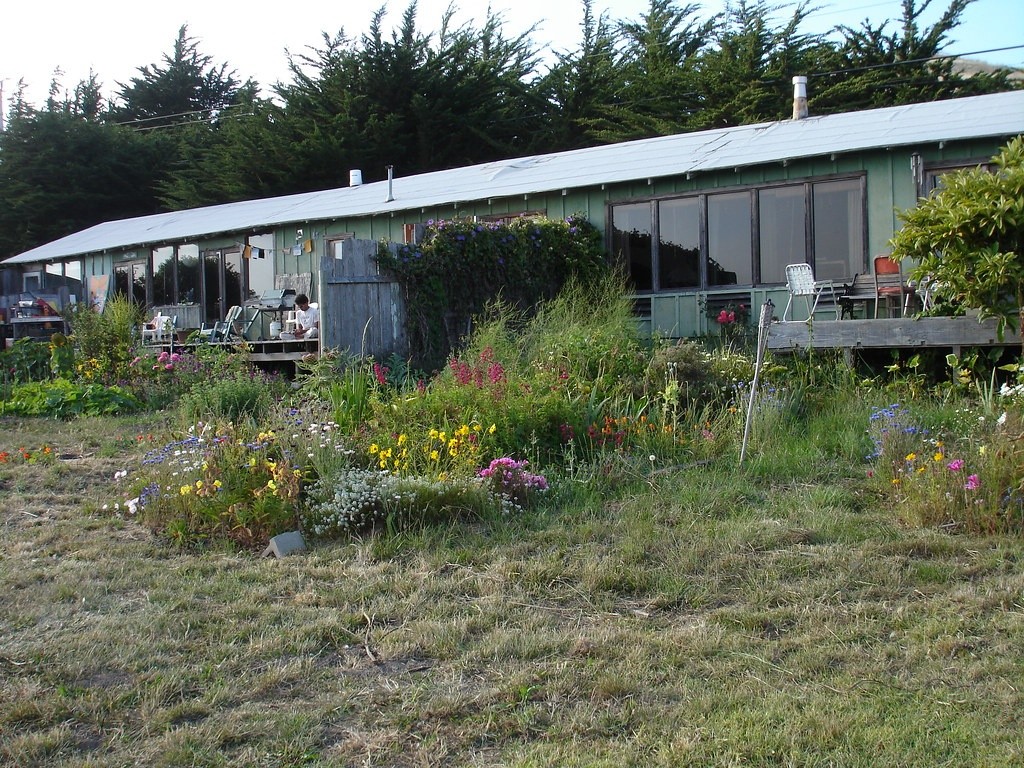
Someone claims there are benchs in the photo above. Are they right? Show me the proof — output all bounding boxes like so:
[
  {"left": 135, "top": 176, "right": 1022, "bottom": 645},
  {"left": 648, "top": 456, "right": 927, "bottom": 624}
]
[{"left": 836, "top": 273, "right": 927, "bottom": 320}]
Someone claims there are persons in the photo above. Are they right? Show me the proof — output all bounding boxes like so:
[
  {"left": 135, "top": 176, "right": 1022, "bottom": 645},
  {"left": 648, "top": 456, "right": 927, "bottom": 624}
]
[{"left": 280, "top": 293, "right": 319, "bottom": 340}]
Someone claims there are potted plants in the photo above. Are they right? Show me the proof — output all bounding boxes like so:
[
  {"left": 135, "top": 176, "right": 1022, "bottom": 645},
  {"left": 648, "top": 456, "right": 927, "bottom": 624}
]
[{"left": 175, "top": 327, "right": 192, "bottom": 344}]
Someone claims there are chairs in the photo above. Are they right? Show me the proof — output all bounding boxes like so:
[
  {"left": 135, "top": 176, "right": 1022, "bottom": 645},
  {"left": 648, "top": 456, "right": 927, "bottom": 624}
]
[
  {"left": 783, "top": 263, "right": 837, "bottom": 322},
  {"left": 197, "top": 306, "right": 242, "bottom": 343},
  {"left": 873, "top": 255, "right": 917, "bottom": 319},
  {"left": 142, "top": 316, "right": 170, "bottom": 344}
]
